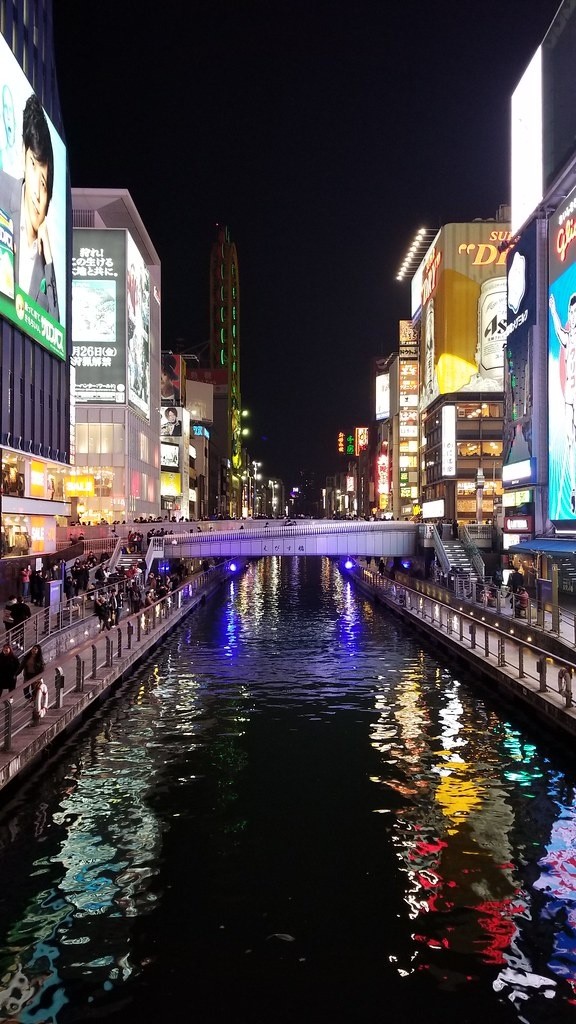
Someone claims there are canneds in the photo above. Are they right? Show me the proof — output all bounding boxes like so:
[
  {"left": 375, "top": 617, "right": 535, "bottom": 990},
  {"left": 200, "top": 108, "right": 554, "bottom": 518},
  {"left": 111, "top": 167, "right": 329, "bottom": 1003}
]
[{"left": 475, "top": 277, "right": 508, "bottom": 385}]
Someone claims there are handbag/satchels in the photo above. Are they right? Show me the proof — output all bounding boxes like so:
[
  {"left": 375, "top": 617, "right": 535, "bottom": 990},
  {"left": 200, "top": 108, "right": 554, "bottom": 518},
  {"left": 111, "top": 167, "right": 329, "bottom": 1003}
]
[{"left": 1, "top": 607, "right": 13, "bottom": 623}]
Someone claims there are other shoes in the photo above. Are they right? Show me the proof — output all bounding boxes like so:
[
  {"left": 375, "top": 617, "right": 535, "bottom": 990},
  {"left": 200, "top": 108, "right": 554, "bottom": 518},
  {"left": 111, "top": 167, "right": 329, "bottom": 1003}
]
[
  {"left": 13, "top": 641, "right": 16, "bottom": 644},
  {"left": 17, "top": 644, "right": 19, "bottom": 647},
  {"left": 9, "top": 698, "right": 13, "bottom": 703},
  {"left": 20, "top": 647, "right": 23, "bottom": 651}
]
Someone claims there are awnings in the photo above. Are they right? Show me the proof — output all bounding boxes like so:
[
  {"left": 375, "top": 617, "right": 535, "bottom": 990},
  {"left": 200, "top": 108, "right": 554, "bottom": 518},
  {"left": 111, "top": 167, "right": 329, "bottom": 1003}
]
[{"left": 507, "top": 539, "right": 576, "bottom": 556}]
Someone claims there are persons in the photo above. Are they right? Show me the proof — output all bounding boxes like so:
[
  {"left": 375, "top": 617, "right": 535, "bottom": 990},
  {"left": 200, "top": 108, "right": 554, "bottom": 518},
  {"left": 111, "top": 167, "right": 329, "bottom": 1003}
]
[
  {"left": 2, "top": 595, "right": 18, "bottom": 644},
  {"left": 417, "top": 517, "right": 458, "bottom": 540},
  {"left": 20, "top": 550, "right": 189, "bottom": 633},
  {"left": 365, "top": 556, "right": 385, "bottom": 579},
  {"left": 16, "top": 645, "right": 44, "bottom": 708},
  {"left": 447, "top": 567, "right": 523, "bottom": 599},
  {"left": 161, "top": 408, "right": 181, "bottom": 435},
  {"left": 515, "top": 587, "right": 529, "bottom": 617},
  {"left": 202, "top": 555, "right": 230, "bottom": 573},
  {"left": 0, "top": 644, "right": 20, "bottom": 704},
  {"left": 0, "top": 94, "right": 60, "bottom": 322},
  {"left": 468, "top": 518, "right": 490, "bottom": 524},
  {"left": 161, "top": 354, "right": 180, "bottom": 399},
  {"left": 4, "top": 596, "right": 31, "bottom": 651},
  {"left": 70, "top": 512, "right": 408, "bottom": 555}
]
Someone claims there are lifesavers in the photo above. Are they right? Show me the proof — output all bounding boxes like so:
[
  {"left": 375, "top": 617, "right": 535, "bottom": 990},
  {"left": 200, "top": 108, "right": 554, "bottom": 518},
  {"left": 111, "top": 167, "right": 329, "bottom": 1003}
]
[
  {"left": 35, "top": 682, "right": 48, "bottom": 719},
  {"left": 189, "top": 584, "right": 193, "bottom": 597},
  {"left": 391, "top": 585, "right": 396, "bottom": 597},
  {"left": 558, "top": 667, "right": 571, "bottom": 697}
]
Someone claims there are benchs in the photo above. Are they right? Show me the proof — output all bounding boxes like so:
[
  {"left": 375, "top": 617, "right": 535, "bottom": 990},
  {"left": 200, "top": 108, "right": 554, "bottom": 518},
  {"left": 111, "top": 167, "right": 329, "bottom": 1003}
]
[{"left": 523, "top": 607, "right": 537, "bottom": 619}]
